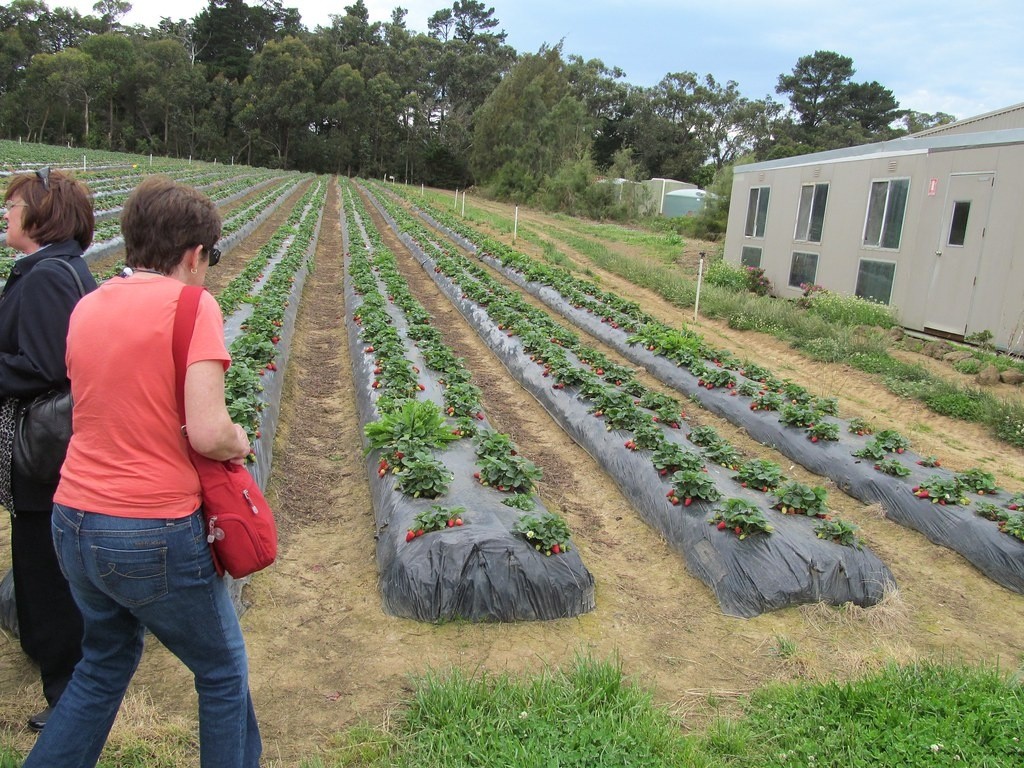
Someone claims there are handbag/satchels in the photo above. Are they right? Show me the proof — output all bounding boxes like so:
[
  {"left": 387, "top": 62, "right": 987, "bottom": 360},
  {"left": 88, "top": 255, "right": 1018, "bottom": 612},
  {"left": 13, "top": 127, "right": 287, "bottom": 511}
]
[
  {"left": 185, "top": 437, "right": 277, "bottom": 579},
  {"left": 0, "top": 389, "right": 72, "bottom": 518}
]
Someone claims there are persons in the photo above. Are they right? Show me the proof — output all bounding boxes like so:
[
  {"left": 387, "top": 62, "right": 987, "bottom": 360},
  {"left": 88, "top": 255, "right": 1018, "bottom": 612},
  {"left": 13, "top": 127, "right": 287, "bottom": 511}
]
[
  {"left": 21, "top": 174, "right": 264, "bottom": 768},
  {"left": 0, "top": 166, "right": 100, "bottom": 733}
]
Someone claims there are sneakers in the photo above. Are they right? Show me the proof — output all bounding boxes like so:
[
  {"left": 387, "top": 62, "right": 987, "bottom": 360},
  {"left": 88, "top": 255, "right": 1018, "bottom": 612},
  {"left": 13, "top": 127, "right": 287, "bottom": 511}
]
[{"left": 29, "top": 708, "right": 50, "bottom": 732}]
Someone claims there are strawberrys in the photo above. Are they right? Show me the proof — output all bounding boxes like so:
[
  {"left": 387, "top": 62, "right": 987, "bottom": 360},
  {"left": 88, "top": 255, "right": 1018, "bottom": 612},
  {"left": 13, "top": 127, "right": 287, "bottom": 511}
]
[{"left": 247, "top": 272, "right": 1018, "bottom": 555}]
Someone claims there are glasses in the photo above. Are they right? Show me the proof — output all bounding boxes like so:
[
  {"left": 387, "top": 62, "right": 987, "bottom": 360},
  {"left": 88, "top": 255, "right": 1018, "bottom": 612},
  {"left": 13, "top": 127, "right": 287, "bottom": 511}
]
[
  {"left": 6, "top": 200, "right": 28, "bottom": 210},
  {"left": 36, "top": 166, "right": 50, "bottom": 192},
  {"left": 209, "top": 248, "right": 221, "bottom": 266}
]
